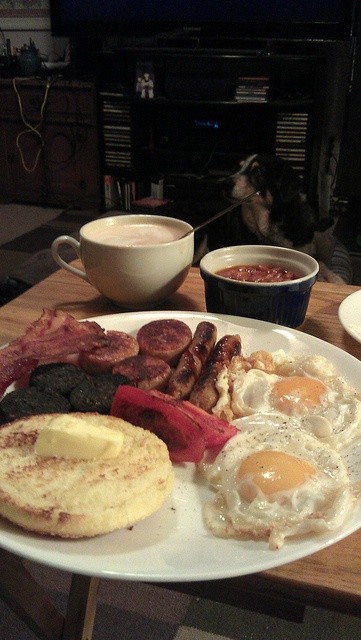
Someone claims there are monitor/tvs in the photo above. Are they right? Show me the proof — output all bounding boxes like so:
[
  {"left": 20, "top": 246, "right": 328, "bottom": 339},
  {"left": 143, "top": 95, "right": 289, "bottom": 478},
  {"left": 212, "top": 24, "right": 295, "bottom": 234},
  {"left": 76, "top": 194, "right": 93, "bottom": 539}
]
[{"left": 48, "top": 0, "right": 360, "bottom": 56}]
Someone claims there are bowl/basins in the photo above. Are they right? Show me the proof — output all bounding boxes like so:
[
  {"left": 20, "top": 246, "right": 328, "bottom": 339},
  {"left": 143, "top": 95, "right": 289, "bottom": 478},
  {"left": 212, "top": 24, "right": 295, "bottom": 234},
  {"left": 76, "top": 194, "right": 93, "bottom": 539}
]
[{"left": 200, "top": 245, "right": 320, "bottom": 329}]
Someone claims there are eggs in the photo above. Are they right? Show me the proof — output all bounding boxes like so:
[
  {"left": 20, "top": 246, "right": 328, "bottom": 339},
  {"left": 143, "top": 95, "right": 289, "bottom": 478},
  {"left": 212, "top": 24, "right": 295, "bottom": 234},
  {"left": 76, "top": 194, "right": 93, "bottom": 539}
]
[
  {"left": 234, "top": 351, "right": 360, "bottom": 442},
  {"left": 204, "top": 427, "right": 351, "bottom": 544}
]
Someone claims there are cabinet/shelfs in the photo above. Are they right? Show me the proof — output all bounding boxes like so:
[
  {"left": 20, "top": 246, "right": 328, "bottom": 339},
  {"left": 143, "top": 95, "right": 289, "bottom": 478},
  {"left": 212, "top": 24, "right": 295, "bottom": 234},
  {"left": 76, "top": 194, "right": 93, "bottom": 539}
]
[
  {"left": 98, "top": 45, "right": 349, "bottom": 224},
  {"left": 0, "top": 81, "right": 100, "bottom": 205}
]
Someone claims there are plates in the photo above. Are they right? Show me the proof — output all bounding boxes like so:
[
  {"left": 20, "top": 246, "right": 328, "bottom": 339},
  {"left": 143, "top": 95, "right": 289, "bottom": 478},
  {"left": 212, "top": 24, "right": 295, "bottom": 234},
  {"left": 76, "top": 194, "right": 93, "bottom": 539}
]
[
  {"left": 1, "top": 308, "right": 360, "bottom": 583},
  {"left": 338, "top": 289, "right": 361, "bottom": 344}
]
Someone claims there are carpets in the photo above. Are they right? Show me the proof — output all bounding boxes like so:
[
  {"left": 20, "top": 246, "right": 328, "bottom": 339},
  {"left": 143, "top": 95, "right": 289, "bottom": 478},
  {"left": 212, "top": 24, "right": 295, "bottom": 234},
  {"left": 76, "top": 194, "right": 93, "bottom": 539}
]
[{"left": 1, "top": 208, "right": 104, "bottom": 285}]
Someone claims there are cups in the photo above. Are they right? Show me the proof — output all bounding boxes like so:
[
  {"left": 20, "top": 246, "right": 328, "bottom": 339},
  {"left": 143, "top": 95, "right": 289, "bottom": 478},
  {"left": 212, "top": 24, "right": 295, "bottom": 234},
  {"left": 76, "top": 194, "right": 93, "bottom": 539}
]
[{"left": 52, "top": 214, "right": 195, "bottom": 310}]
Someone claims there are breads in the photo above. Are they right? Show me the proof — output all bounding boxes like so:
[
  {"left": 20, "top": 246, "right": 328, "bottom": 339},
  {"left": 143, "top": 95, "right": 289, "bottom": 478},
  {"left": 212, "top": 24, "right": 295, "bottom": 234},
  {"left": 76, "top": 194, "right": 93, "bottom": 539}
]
[{"left": 1, "top": 412, "right": 175, "bottom": 536}]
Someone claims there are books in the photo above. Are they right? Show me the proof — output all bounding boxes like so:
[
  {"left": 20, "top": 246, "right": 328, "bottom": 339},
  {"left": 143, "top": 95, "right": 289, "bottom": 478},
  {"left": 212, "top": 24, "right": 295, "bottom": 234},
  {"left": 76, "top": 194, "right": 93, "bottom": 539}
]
[
  {"left": 275, "top": 112, "right": 308, "bottom": 179},
  {"left": 99, "top": 91, "right": 133, "bottom": 168},
  {"left": 104, "top": 174, "right": 136, "bottom": 211},
  {"left": 234, "top": 77, "right": 272, "bottom": 103}
]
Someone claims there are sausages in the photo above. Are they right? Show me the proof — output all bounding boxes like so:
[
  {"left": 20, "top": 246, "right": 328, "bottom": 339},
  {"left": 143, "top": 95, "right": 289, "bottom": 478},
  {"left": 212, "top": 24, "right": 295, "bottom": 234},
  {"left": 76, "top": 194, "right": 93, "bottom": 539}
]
[
  {"left": 168, "top": 321, "right": 219, "bottom": 399},
  {"left": 191, "top": 334, "right": 245, "bottom": 410}
]
